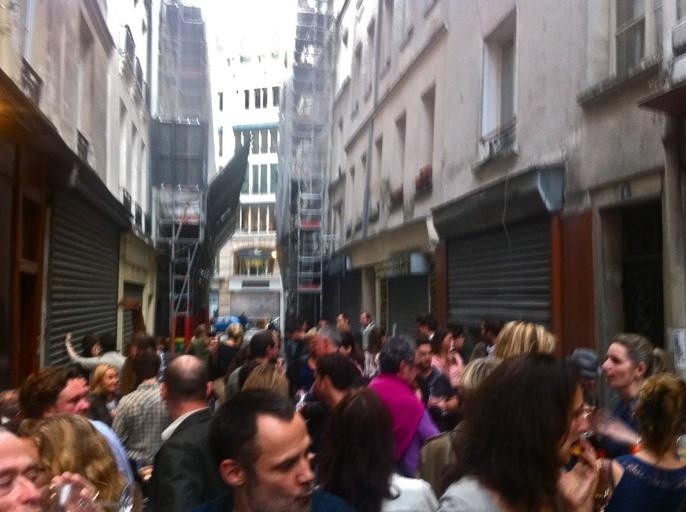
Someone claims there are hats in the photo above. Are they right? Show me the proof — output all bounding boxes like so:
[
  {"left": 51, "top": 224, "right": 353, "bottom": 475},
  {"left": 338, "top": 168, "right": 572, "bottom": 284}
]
[{"left": 560, "top": 348, "right": 603, "bottom": 383}]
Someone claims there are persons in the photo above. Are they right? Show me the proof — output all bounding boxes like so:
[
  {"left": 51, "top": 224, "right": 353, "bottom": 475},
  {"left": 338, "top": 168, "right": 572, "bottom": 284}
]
[
  {"left": 115, "top": 331, "right": 157, "bottom": 407},
  {"left": 286, "top": 319, "right": 316, "bottom": 386},
  {"left": 17, "top": 413, "right": 143, "bottom": 512},
  {"left": 0, "top": 425, "right": 97, "bottom": 512},
  {"left": 238, "top": 359, "right": 262, "bottom": 388},
  {"left": 209, "top": 323, "right": 245, "bottom": 383},
  {"left": 430, "top": 329, "right": 466, "bottom": 390},
  {"left": 337, "top": 311, "right": 365, "bottom": 376},
  {"left": 410, "top": 339, "right": 464, "bottom": 434},
  {"left": 313, "top": 387, "right": 438, "bottom": 511},
  {"left": 240, "top": 319, "right": 266, "bottom": 356},
  {"left": 184, "top": 324, "right": 209, "bottom": 367},
  {"left": 488, "top": 321, "right": 559, "bottom": 362},
  {"left": 89, "top": 363, "right": 124, "bottom": 428},
  {"left": 316, "top": 325, "right": 341, "bottom": 354},
  {"left": 137, "top": 354, "right": 233, "bottom": 512},
  {"left": 586, "top": 372, "right": 685, "bottom": 512},
  {"left": 448, "top": 323, "right": 467, "bottom": 352},
  {"left": 227, "top": 330, "right": 289, "bottom": 405},
  {"left": 307, "top": 319, "right": 328, "bottom": 336},
  {"left": 369, "top": 339, "right": 438, "bottom": 480},
  {"left": 353, "top": 311, "right": 385, "bottom": 378},
  {"left": 334, "top": 330, "right": 365, "bottom": 379},
  {"left": 193, "top": 387, "right": 359, "bottom": 512},
  {"left": 418, "top": 314, "right": 447, "bottom": 355},
  {"left": 439, "top": 352, "right": 600, "bottom": 512},
  {"left": 242, "top": 363, "right": 288, "bottom": 403},
  {"left": 203, "top": 332, "right": 226, "bottom": 405},
  {"left": 313, "top": 352, "right": 355, "bottom": 410},
  {"left": 1, "top": 389, "right": 19, "bottom": 437},
  {"left": 112, "top": 352, "right": 172, "bottom": 500},
  {"left": 238, "top": 311, "right": 248, "bottom": 331},
  {"left": 78, "top": 333, "right": 102, "bottom": 380},
  {"left": 19, "top": 366, "right": 144, "bottom": 512},
  {"left": 66, "top": 333, "right": 128, "bottom": 375},
  {"left": 420, "top": 355, "right": 507, "bottom": 501},
  {"left": 600, "top": 335, "right": 668, "bottom": 458},
  {"left": 562, "top": 347, "right": 600, "bottom": 471}
]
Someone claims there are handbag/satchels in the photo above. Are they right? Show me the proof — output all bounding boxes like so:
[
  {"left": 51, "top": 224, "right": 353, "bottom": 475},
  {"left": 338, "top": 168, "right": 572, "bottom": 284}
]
[{"left": 593, "top": 459, "right": 613, "bottom": 511}]
